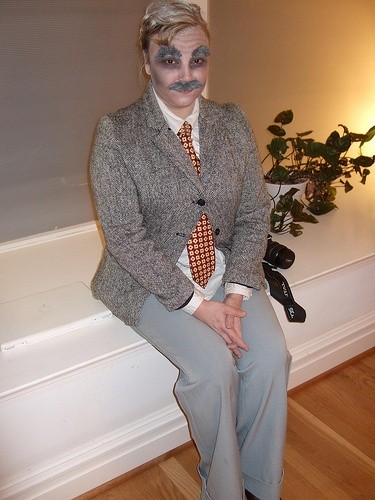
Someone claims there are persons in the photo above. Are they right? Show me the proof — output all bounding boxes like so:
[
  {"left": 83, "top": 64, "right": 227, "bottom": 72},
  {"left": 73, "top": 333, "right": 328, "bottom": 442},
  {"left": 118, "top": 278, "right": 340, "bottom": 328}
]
[{"left": 88, "top": 1, "right": 292, "bottom": 500}]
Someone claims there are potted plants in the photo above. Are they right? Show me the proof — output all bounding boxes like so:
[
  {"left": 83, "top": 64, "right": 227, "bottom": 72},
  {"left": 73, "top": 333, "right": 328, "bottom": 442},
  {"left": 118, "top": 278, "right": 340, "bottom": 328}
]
[{"left": 261, "top": 109, "right": 375, "bottom": 238}]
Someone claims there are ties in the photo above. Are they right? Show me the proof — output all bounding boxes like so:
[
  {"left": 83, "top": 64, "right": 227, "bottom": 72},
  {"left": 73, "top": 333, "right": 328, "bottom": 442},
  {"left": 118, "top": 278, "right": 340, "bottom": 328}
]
[{"left": 178, "top": 121, "right": 216, "bottom": 289}]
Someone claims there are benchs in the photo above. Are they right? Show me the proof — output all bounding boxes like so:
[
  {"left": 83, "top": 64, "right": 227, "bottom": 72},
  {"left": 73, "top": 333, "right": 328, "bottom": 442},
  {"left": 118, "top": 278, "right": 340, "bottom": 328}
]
[{"left": 0, "top": 164, "right": 375, "bottom": 500}]
